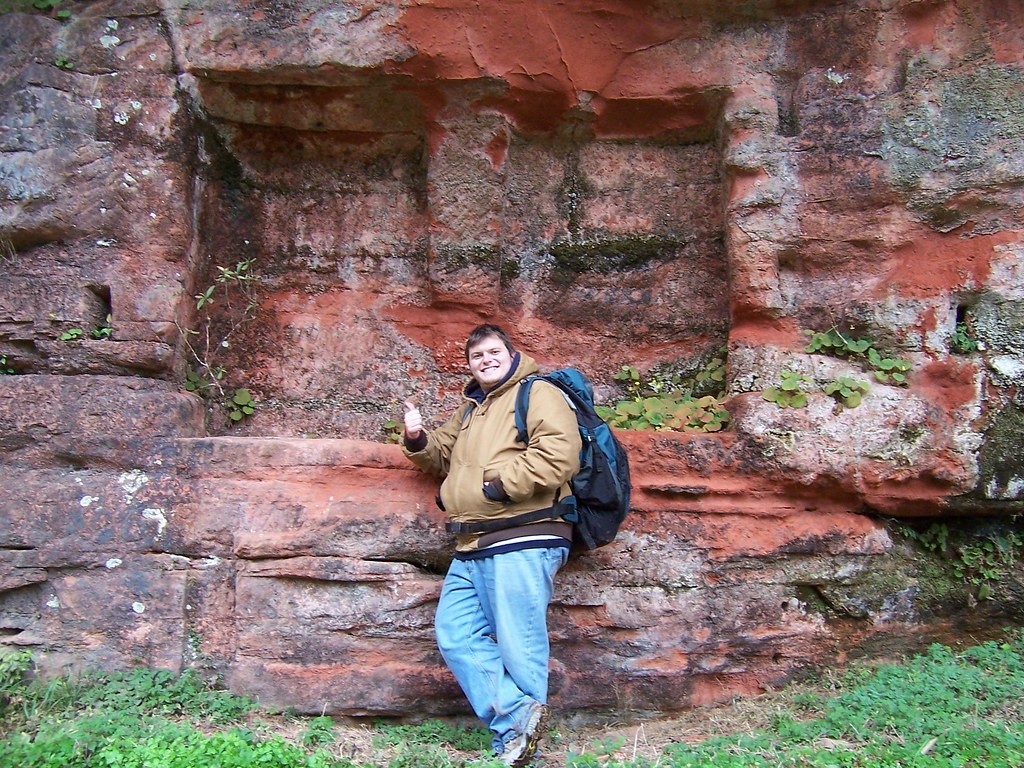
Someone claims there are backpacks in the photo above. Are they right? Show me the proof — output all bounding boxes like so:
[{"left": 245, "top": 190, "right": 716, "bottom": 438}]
[{"left": 462, "top": 368, "right": 633, "bottom": 549}]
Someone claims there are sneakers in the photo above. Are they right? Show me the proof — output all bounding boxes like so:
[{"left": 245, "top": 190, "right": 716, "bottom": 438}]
[
  {"left": 462, "top": 752, "right": 498, "bottom": 768},
  {"left": 498, "top": 701, "right": 551, "bottom": 768}
]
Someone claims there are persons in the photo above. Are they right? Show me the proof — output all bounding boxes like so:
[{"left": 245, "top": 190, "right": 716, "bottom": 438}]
[{"left": 398, "top": 323, "right": 582, "bottom": 768}]
